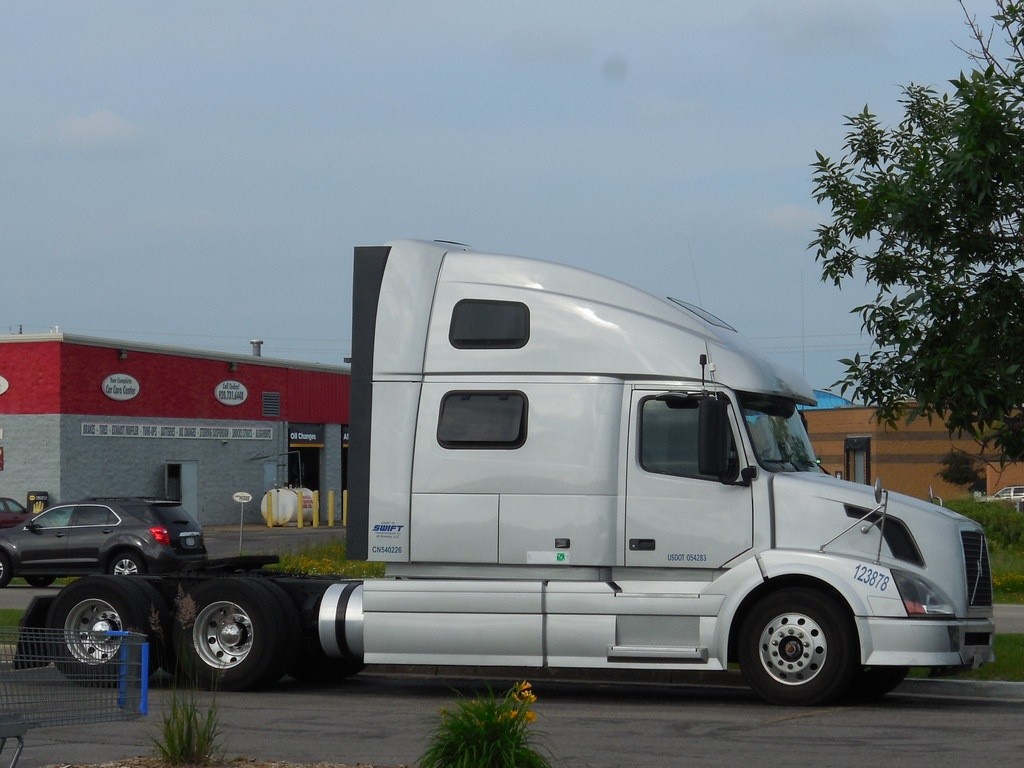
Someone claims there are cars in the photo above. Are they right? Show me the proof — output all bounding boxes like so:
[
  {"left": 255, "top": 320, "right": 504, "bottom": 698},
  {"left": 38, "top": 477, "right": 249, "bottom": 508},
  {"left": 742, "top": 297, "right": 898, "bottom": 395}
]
[
  {"left": 0, "top": 497, "right": 38, "bottom": 528},
  {"left": 977, "top": 484, "right": 1024, "bottom": 503}
]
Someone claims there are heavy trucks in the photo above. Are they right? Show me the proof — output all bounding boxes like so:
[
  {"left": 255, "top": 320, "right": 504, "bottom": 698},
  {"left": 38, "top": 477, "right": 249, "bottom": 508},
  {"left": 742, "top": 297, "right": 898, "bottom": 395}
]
[{"left": 12, "top": 238, "right": 997, "bottom": 707}]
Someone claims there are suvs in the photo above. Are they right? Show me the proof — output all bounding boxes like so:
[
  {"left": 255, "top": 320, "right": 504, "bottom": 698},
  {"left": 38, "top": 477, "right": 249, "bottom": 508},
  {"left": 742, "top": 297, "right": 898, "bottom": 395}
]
[{"left": 0, "top": 496, "right": 209, "bottom": 589}]
